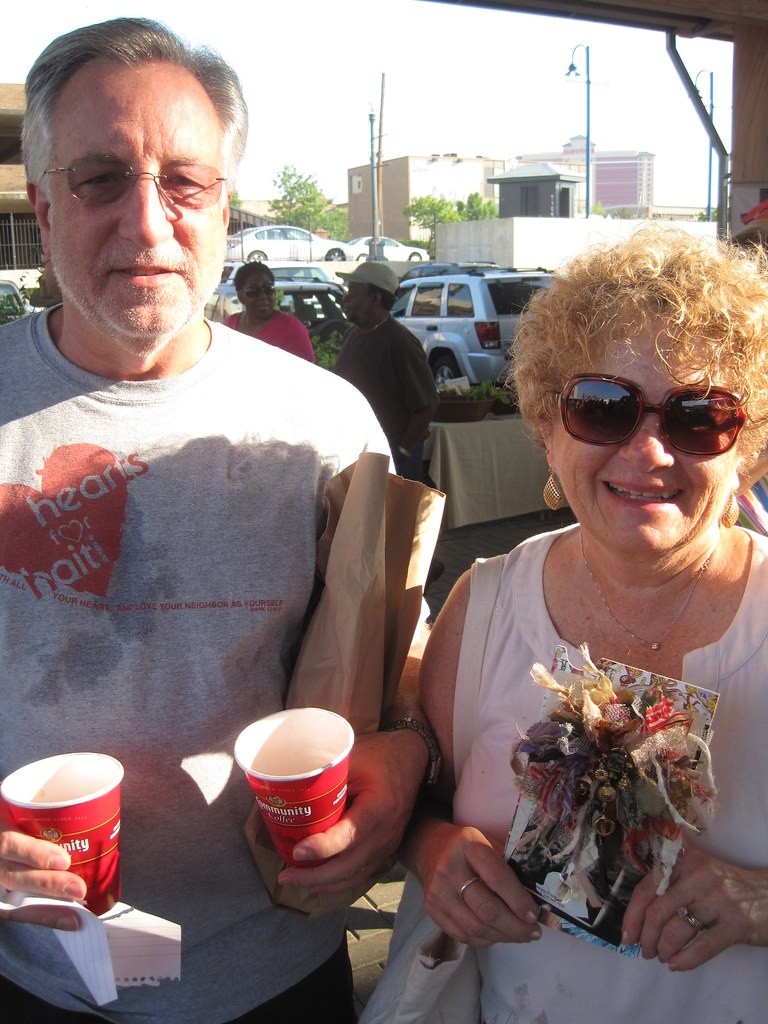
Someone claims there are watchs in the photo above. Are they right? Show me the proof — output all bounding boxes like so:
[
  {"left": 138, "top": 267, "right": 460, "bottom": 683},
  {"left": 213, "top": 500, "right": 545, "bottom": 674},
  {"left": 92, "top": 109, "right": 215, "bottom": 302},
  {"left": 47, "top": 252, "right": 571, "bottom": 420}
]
[{"left": 379, "top": 716, "right": 442, "bottom": 792}]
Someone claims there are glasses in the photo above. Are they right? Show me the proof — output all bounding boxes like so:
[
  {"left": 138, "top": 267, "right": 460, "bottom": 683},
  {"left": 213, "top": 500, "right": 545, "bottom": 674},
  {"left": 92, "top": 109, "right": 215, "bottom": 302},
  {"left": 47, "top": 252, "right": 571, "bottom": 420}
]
[
  {"left": 554, "top": 373, "right": 747, "bottom": 455},
  {"left": 242, "top": 283, "right": 275, "bottom": 298},
  {"left": 45, "top": 155, "right": 228, "bottom": 210}
]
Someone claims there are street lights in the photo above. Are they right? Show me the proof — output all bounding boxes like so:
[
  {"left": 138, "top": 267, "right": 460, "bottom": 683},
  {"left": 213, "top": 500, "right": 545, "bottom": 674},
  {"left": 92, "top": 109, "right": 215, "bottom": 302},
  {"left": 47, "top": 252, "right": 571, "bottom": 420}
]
[
  {"left": 565, "top": 44, "right": 591, "bottom": 217},
  {"left": 688, "top": 69, "right": 715, "bottom": 223},
  {"left": 368, "top": 106, "right": 387, "bottom": 240}
]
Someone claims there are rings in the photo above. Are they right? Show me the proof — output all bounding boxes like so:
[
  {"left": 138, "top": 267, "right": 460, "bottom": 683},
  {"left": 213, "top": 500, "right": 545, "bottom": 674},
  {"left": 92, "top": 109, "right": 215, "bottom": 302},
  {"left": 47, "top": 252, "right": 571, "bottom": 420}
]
[
  {"left": 458, "top": 877, "right": 482, "bottom": 900},
  {"left": 676, "top": 905, "right": 705, "bottom": 931}
]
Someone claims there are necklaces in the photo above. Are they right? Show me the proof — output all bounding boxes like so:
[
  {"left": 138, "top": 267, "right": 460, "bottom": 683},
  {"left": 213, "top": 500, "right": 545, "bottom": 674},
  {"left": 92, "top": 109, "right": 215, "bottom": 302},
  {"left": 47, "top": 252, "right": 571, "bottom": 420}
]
[
  {"left": 372, "top": 316, "right": 389, "bottom": 330},
  {"left": 579, "top": 527, "right": 714, "bottom": 650}
]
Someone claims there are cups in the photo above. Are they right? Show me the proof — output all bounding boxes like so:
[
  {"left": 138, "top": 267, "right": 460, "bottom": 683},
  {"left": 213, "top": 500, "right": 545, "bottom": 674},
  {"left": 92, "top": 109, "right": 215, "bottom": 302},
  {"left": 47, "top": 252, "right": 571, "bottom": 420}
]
[
  {"left": 234, "top": 708, "right": 355, "bottom": 868},
  {"left": 0, "top": 751, "right": 125, "bottom": 917}
]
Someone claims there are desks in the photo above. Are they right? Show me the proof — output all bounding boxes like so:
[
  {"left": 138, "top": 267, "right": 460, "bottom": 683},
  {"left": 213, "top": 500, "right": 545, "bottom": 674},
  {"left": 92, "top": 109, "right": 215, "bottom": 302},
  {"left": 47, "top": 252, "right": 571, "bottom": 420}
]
[{"left": 422, "top": 412, "right": 569, "bottom": 530}]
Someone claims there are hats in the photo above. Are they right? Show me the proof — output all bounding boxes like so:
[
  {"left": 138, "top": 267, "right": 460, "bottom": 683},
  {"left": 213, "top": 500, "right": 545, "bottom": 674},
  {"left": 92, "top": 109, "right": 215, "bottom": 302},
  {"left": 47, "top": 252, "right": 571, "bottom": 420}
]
[{"left": 335, "top": 261, "right": 399, "bottom": 296}]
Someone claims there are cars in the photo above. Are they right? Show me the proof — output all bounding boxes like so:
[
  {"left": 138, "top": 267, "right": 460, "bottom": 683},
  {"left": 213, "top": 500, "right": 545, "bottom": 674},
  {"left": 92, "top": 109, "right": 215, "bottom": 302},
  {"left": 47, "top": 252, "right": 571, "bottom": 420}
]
[
  {"left": 345, "top": 237, "right": 432, "bottom": 262},
  {"left": 0, "top": 281, "right": 34, "bottom": 325},
  {"left": 225, "top": 225, "right": 350, "bottom": 261}
]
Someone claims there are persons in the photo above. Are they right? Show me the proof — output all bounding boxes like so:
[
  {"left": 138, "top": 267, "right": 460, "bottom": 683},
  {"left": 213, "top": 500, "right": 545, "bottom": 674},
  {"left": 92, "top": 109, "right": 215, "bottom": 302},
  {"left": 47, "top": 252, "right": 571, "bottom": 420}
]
[
  {"left": 0, "top": 21, "right": 434, "bottom": 1024},
  {"left": 227, "top": 222, "right": 768, "bottom": 1023}
]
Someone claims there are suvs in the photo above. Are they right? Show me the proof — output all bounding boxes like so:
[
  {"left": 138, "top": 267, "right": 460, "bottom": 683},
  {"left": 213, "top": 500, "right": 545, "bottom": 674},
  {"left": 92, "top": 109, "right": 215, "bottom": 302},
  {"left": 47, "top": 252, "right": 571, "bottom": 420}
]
[
  {"left": 206, "top": 261, "right": 354, "bottom": 343},
  {"left": 390, "top": 262, "right": 568, "bottom": 388}
]
